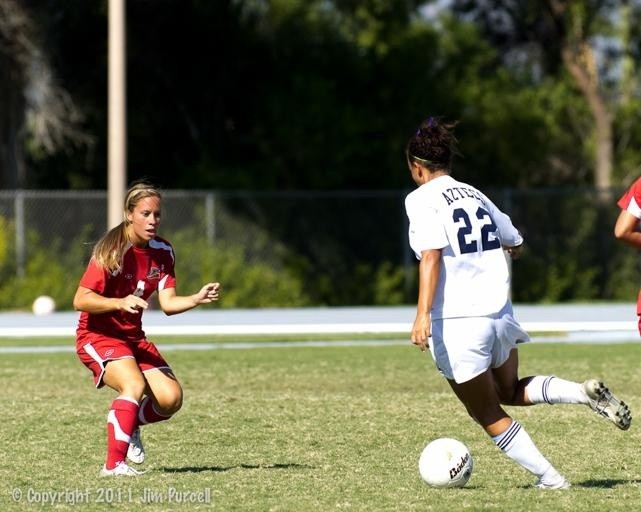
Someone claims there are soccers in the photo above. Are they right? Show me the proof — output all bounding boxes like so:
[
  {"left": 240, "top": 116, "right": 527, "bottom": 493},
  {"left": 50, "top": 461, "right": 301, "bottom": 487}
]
[
  {"left": 418, "top": 437, "right": 473, "bottom": 490},
  {"left": 30, "top": 296, "right": 56, "bottom": 316}
]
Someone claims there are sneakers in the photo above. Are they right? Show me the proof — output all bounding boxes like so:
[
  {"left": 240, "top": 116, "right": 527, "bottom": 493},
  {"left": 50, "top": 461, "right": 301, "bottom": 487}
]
[
  {"left": 99, "top": 462, "right": 145, "bottom": 478},
  {"left": 126, "top": 429, "right": 145, "bottom": 464},
  {"left": 580, "top": 378, "right": 632, "bottom": 431}
]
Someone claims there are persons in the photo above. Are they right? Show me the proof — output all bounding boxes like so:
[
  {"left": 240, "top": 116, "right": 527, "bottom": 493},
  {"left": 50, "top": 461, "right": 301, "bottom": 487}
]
[
  {"left": 71, "top": 182, "right": 221, "bottom": 477},
  {"left": 613, "top": 174, "right": 641, "bottom": 336},
  {"left": 404, "top": 116, "right": 634, "bottom": 491}
]
